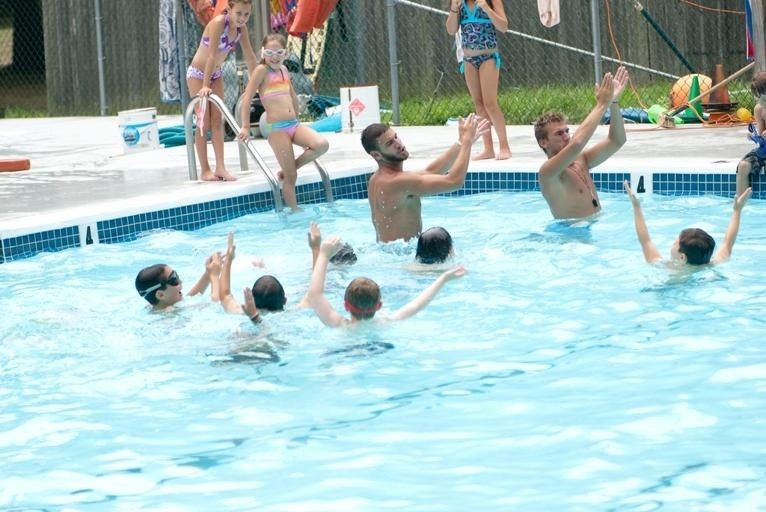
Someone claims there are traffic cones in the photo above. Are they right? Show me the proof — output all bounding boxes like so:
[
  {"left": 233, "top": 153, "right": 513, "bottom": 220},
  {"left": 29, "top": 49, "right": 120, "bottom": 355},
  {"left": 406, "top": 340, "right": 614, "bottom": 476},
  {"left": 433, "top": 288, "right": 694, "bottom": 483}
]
[{"left": 700, "top": 64, "right": 739, "bottom": 108}]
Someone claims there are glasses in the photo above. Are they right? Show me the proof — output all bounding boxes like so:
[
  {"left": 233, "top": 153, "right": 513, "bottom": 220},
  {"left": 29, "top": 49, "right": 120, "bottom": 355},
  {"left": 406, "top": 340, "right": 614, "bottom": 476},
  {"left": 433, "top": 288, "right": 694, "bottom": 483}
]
[
  {"left": 140, "top": 270, "right": 181, "bottom": 299},
  {"left": 262, "top": 49, "right": 287, "bottom": 56}
]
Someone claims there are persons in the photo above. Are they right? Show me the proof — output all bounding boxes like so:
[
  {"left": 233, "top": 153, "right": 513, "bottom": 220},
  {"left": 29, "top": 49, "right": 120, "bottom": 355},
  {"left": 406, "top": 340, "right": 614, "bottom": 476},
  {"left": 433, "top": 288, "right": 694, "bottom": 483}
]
[
  {"left": 185, "top": 0, "right": 259, "bottom": 181},
  {"left": 533, "top": 64, "right": 630, "bottom": 221},
  {"left": 623, "top": 179, "right": 753, "bottom": 270},
  {"left": 236, "top": 34, "right": 329, "bottom": 215},
  {"left": 736, "top": 72, "right": 766, "bottom": 198},
  {"left": 445, "top": 0, "right": 511, "bottom": 160},
  {"left": 360, "top": 111, "right": 491, "bottom": 242}
]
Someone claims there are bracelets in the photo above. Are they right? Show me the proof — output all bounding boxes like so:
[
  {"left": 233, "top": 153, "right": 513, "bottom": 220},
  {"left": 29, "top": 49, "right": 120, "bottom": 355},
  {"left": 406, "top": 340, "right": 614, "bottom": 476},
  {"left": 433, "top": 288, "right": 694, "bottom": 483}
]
[
  {"left": 611, "top": 99, "right": 621, "bottom": 103},
  {"left": 454, "top": 140, "right": 462, "bottom": 148},
  {"left": 450, "top": 8, "right": 460, "bottom": 14}
]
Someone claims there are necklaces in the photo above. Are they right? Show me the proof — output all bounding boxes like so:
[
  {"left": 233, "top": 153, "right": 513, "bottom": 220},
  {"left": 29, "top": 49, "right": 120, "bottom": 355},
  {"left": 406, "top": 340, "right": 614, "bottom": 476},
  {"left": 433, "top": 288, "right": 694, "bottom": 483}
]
[{"left": 569, "top": 162, "right": 600, "bottom": 207}]
